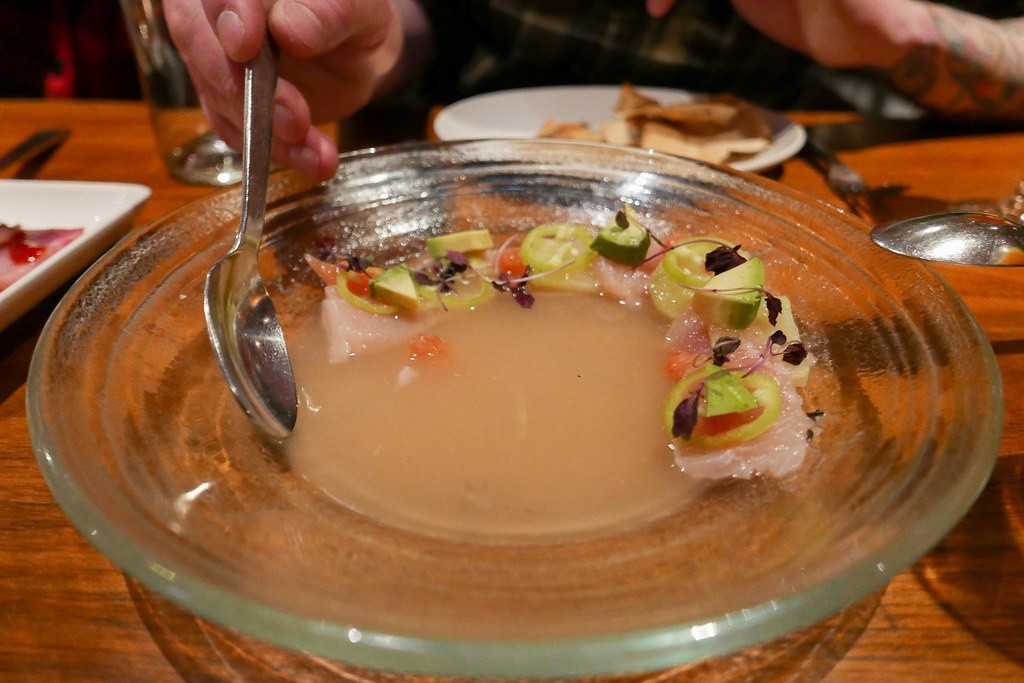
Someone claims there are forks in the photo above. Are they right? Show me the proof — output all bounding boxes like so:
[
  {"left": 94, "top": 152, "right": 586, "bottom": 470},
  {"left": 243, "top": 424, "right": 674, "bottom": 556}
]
[{"left": 803, "top": 138, "right": 911, "bottom": 197}]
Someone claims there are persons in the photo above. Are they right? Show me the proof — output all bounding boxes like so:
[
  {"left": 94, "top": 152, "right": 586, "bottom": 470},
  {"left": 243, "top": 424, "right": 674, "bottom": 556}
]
[{"left": 143, "top": 0, "right": 1024, "bottom": 201}]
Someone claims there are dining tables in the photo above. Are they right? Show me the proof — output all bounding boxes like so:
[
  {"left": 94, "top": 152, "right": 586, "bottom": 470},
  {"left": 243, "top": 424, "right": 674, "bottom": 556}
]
[{"left": 0, "top": 96, "right": 1024, "bottom": 683}]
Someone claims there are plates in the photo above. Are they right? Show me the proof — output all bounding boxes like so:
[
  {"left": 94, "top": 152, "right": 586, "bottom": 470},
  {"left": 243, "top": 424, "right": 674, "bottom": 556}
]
[
  {"left": 431, "top": 88, "right": 808, "bottom": 170},
  {"left": 0, "top": 175, "right": 151, "bottom": 320},
  {"left": 23, "top": 133, "right": 1005, "bottom": 671}
]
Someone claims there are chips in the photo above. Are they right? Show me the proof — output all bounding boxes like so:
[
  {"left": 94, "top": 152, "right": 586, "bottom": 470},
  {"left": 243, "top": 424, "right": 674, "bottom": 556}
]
[{"left": 534, "top": 79, "right": 772, "bottom": 163}]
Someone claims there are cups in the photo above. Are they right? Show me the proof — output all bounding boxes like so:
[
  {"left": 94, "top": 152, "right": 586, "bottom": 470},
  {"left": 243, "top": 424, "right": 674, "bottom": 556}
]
[{"left": 119, "top": 0, "right": 246, "bottom": 184}]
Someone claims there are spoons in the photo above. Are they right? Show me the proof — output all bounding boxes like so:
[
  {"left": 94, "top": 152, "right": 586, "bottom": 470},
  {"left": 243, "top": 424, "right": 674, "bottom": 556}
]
[
  {"left": 868, "top": 212, "right": 1024, "bottom": 265},
  {"left": 205, "top": 29, "right": 297, "bottom": 441}
]
